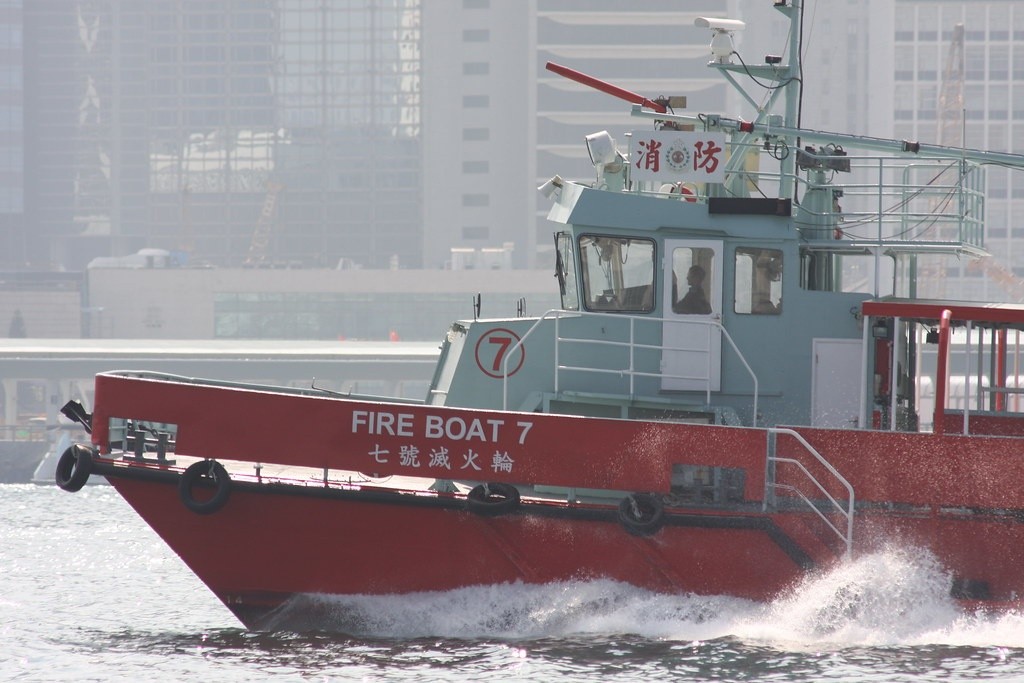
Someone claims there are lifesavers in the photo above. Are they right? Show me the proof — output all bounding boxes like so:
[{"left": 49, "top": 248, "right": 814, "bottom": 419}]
[{"left": 669, "top": 187, "right": 697, "bottom": 203}]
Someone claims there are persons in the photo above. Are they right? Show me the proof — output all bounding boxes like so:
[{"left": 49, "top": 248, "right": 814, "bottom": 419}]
[{"left": 673, "top": 266, "right": 709, "bottom": 314}]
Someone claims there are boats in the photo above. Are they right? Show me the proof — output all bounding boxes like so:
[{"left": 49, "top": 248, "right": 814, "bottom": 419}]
[{"left": 54, "top": 0, "right": 1020, "bottom": 627}]
